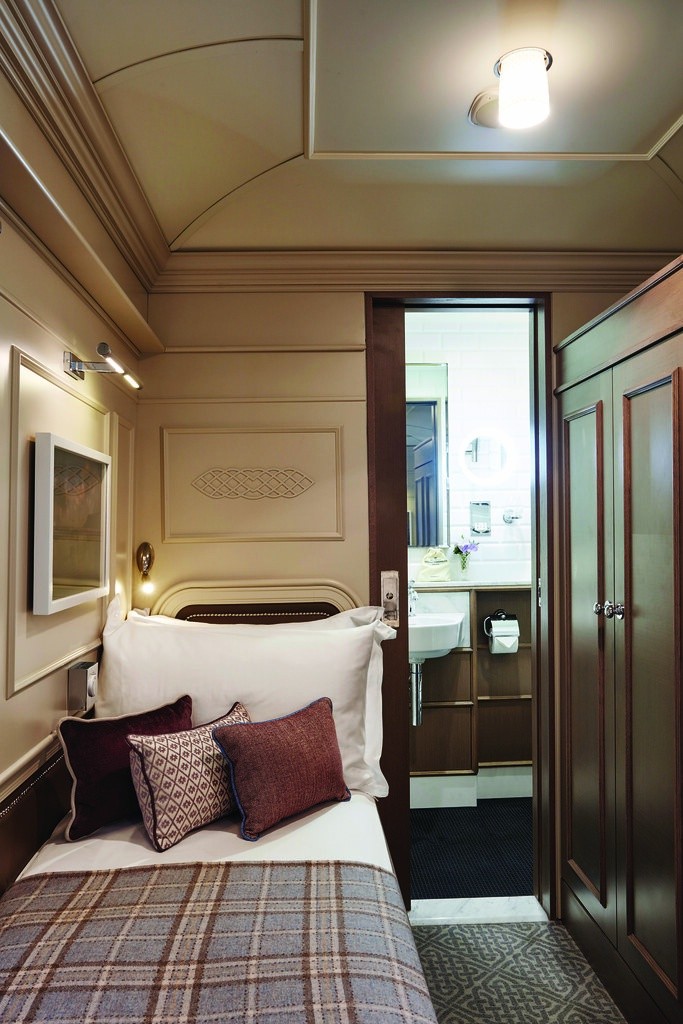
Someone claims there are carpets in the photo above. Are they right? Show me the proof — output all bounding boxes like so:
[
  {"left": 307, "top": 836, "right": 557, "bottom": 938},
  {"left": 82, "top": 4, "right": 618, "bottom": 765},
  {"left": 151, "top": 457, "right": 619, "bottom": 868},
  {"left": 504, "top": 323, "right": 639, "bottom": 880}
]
[
  {"left": 410, "top": 798, "right": 534, "bottom": 901},
  {"left": 410, "top": 922, "right": 628, "bottom": 1024}
]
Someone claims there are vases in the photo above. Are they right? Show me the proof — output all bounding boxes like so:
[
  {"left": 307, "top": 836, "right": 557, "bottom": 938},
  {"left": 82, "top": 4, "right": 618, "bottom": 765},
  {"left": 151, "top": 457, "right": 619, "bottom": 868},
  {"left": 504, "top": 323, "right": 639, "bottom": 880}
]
[{"left": 456, "top": 552, "right": 473, "bottom": 581}]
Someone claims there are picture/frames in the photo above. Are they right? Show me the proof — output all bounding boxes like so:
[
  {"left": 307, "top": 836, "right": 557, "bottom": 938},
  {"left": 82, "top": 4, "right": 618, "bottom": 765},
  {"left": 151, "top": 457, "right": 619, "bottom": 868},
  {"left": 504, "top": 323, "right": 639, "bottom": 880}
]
[{"left": 32, "top": 431, "right": 112, "bottom": 615}]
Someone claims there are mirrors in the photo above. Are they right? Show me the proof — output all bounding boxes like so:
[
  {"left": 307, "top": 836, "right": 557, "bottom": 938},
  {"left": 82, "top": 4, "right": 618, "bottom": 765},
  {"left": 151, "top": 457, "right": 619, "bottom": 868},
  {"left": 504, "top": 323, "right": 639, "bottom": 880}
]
[{"left": 406, "top": 362, "right": 448, "bottom": 551}]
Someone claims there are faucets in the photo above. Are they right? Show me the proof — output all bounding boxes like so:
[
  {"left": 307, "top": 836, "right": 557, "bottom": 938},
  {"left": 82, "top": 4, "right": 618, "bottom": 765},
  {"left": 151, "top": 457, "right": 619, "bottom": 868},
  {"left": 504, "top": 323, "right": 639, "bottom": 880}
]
[{"left": 408, "top": 588, "right": 419, "bottom": 618}]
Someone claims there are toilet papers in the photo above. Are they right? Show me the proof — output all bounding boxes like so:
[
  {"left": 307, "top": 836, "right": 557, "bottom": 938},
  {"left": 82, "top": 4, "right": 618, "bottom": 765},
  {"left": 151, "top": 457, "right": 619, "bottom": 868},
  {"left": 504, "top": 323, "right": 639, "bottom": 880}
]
[{"left": 489, "top": 620, "right": 520, "bottom": 653}]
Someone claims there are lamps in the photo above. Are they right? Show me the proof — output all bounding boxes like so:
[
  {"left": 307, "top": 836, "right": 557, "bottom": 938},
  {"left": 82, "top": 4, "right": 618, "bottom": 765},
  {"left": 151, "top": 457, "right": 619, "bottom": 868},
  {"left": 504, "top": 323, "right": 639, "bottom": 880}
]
[
  {"left": 63, "top": 342, "right": 145, "bottom": 391},
  {"left": 137, "top": 542, "right": 155, "bottom": 593},
  {"left": 492, "top": 0, "right": 559, "bottom": 131}
]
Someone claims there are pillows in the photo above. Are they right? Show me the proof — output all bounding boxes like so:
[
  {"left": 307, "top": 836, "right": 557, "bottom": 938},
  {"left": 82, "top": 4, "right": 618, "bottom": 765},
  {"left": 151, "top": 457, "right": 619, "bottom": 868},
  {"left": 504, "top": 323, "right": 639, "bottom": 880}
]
[
  {"left": 211, "top": 698, "right": 351, "bottom": 842},
  {"left": 56, "top": 694, "right": 193, "bottom": 843},
  {"left": 124, "top": 702, "right": 252, "bottom": 853},
  {"left": 94, "top": 594, "right": 396, "bottom": 798}
]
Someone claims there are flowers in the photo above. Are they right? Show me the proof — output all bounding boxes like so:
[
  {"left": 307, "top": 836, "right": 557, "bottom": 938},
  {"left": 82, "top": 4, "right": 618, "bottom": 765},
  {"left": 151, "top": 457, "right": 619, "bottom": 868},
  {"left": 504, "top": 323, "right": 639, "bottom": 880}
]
[{"left": 453, "top": 534, "right": 480, "bottom": 571}]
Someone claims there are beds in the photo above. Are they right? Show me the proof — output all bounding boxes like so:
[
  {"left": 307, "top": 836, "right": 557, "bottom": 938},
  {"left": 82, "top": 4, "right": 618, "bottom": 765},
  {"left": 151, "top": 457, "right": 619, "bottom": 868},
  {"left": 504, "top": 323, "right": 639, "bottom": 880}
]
[{"left": 0, "top": 579, "right": 439, "bottom": 1024}]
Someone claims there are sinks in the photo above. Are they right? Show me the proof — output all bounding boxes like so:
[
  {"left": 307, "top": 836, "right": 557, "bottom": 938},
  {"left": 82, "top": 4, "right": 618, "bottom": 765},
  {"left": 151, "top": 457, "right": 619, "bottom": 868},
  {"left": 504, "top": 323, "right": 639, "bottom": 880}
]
[{"left": 407, "top": 613, "right": 465, "bottom": 663}]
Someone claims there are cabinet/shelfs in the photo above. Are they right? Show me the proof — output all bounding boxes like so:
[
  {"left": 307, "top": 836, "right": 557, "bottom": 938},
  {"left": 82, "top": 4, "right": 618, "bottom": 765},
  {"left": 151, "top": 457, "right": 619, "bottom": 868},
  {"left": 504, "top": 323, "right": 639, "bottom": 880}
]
[
  {"left": 553, "top": 256, "right": 683, "bottom": 1024},
  {"left": 412, "top": 438, "right": 439, "bottom": 546}
]
